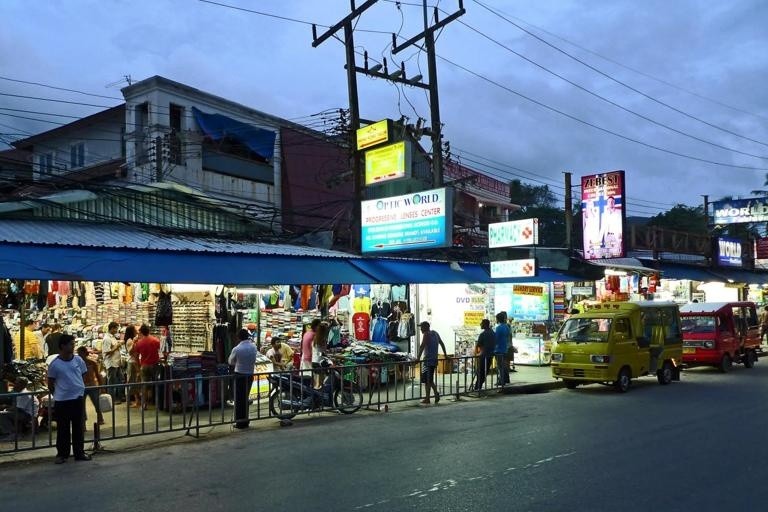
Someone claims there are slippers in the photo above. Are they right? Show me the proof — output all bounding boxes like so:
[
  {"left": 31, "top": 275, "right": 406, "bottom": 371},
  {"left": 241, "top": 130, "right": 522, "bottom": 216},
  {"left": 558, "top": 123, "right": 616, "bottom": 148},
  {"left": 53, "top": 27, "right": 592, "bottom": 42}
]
[{"left": 421, "top": 392, "right": 440, "bottom": 404}]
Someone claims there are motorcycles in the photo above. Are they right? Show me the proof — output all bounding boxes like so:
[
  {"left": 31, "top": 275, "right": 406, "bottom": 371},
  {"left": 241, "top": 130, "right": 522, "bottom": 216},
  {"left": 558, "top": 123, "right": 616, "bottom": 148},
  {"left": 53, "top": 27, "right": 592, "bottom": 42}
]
[{"left": 264, "top": 355, "right": 363, "bottom": 420}]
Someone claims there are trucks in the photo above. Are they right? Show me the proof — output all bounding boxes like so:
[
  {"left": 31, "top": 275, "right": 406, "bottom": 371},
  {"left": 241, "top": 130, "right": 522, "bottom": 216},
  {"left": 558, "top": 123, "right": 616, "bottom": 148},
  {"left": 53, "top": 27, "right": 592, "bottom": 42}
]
[
  {"left": 674, "top": 301, "right": 763, "bottom": 372},
  {"left": 548, "top": 298, "right": 684, "bottom": 390}
]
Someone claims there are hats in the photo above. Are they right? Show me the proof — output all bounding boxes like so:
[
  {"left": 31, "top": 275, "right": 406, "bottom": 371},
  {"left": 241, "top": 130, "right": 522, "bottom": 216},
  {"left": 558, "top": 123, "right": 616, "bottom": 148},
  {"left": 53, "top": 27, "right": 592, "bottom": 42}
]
[{"left": 418, "top": 321, "right": 430, "bottom": 327}]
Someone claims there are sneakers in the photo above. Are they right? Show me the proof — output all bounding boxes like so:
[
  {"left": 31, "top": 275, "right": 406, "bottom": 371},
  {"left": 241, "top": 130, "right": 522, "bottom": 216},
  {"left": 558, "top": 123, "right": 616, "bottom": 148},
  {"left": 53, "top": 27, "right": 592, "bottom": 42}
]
[
  {"left": 54, "top": 453, "right": 92, "bottom": 465},
  {"left": 474, "top": 367, "right": 517, "bottom": 391},
  {"left": 233, "top": 423, "right": 250, "bottom": 429},
  {"left": 84, "top": 394, "right": 161, "bottom": 425}
]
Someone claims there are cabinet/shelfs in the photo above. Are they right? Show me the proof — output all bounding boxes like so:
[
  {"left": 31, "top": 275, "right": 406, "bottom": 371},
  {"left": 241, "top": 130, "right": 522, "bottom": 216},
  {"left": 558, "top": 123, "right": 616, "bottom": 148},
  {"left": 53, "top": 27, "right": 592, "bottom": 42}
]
[
  {"left": 512, "top": 332, "right": 559, "bottom": 368},
  {"left": 247, "top": 349, "right": 277, "bottom": 401},
  {"left": 153, "top": 367, "right": 227, "bottom": 414},
  {"left": 451, "top": 324, "right": 479, "bottom": 376}
]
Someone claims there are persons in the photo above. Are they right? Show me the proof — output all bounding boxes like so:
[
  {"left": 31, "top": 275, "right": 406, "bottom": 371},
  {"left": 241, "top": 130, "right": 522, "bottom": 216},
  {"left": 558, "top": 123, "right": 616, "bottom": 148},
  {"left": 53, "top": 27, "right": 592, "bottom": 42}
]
[
  {"left": 226, "top": 328, "right": 257, "bottom": 430},
  {"left": 582, "top": 200, "right": 600, "bottom": 244},
  {"left": 42, "top": 334, "right": 92, "bottom": 466},
  {"left": 757, "top": 304, "right": 768, "bottom": 345},
  {"left": 12, "top": 318, "right": 70, "bottom": 362},
  {"left": 280, "top": 341, "right": 295, "bottom": 368},
  {"left": 408, "top": 321, "right": 449, "bottom": 404},
  {"left": 265, "top": 336, "right": 285, "bottom": 371},
  {"left": 299, "top": 316, "right": 343, "bottom": 390},
  {"left": 471, "top": 310, "right": 514, "bottom": 392},
  {"left": 75, "top": 346, "right": 105, "bottom": 428},
  {"left": 101, "top": 323, "right": 160, "bottom": 411},
  {"left": 601, "top": 197, "right": 622, "bottom": 243},
  {"left": 0, "top": 375, "right": 39, "bottom": 441}
]
